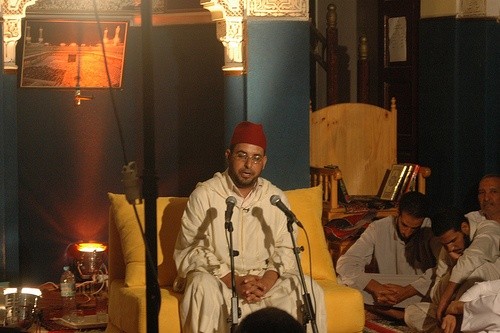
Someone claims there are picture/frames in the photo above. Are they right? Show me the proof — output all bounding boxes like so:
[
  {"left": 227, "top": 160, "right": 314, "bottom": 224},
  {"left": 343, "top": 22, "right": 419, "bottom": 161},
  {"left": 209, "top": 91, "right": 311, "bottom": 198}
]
[{"left": 19, "top": 19, "right": 129, "bottom": 92}]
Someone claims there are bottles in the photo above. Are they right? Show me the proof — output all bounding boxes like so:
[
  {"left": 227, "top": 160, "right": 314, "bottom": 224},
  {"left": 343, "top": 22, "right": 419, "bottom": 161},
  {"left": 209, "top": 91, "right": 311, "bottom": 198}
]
[{"left": 61, "top": 266, "right": 77, "bottom": 322}]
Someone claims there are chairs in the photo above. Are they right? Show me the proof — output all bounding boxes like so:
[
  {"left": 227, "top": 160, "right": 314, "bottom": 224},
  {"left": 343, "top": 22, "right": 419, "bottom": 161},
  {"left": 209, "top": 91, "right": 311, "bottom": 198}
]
[{"left": 309, "top": 95, "right": 431, "bottom": 232}]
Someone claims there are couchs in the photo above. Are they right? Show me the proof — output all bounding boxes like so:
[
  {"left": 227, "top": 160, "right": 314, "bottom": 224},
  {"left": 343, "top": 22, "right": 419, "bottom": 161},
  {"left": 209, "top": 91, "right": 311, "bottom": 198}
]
[{"left": 103, "top": 192, "right": 366, "bottom": 332}]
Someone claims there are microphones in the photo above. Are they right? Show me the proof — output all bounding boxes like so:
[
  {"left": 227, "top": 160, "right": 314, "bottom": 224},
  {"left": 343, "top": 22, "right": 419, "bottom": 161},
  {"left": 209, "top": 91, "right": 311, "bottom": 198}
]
[
  {"left": 225, "top": 195, "right": 236, "bottom": 223},
  {"left": 270, "top": 194, "right": 304, "bottom": 229}
]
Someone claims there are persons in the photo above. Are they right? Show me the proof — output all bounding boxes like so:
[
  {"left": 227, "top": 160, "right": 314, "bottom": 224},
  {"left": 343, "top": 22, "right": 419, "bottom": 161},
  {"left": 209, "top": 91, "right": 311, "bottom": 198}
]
[
  {"left": 335, "top": 172, "right": 500, "bottom": 333},
  {"left": 172, "top": 120, "right": 325, "bottom": 333}
]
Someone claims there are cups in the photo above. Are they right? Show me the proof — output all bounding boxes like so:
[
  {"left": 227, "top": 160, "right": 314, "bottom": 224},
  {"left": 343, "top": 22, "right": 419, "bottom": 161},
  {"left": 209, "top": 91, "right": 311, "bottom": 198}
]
[
  {"left": 96, "top": 294, "right": 108, "bottom": 322},
  {"left": 68, "top": 309, "right": 89, "bottom": 323}
]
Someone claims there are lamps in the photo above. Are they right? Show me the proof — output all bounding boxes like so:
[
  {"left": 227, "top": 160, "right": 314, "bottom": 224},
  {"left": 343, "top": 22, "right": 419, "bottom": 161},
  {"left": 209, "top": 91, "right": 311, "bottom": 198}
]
[
  {"left": 2, "top": 287, "right": 42, "bottom": 331},
  {"left": 75, "top": 239, "right": 106, "bottom": 274}
]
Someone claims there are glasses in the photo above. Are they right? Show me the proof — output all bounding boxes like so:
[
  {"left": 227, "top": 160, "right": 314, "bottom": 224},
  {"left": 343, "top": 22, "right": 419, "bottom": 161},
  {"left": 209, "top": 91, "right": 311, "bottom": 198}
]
[{"left": 231, "top": 152, "right": 265, "bottom": 164}]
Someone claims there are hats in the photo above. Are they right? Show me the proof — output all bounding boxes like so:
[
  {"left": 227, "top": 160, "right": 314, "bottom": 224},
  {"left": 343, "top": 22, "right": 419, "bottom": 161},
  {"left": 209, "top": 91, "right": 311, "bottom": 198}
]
[{"left": 230, "top": 121, "right": 266, "bottom": 150}]
